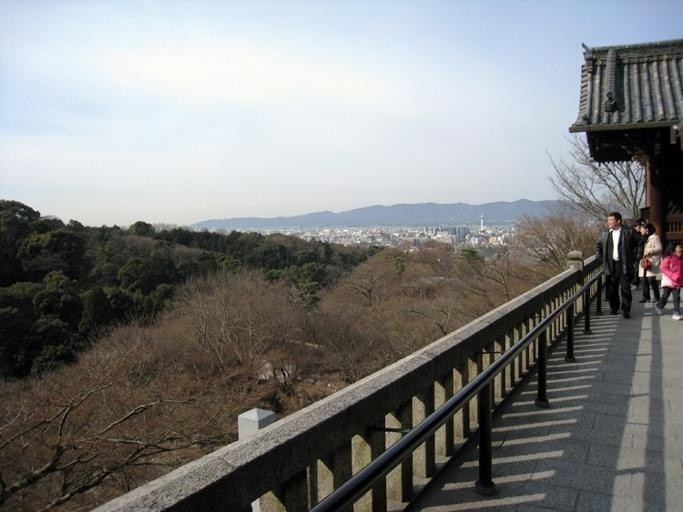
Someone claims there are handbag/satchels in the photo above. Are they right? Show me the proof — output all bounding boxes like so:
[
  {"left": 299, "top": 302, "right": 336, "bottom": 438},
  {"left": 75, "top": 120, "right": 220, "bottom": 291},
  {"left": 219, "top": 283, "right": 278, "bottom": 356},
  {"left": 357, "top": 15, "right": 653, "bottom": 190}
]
[{"left": 640, "top": 255, "right": 653, "bottom": 271}]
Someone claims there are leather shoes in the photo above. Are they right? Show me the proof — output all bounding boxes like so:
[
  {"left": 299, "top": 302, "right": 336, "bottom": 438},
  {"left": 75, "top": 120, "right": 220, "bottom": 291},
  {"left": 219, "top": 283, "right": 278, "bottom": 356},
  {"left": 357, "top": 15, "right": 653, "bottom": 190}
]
[
  {"left": 609, "top": 308, "right": 617, "bottom": 315},
  {"left": 623, "top": 310, "right": 630, "bottom": 319}
]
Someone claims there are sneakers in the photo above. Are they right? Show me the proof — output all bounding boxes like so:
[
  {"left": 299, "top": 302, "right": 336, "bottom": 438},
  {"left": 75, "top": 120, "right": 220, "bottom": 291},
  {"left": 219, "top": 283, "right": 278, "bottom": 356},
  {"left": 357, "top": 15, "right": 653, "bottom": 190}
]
[
  {"left": 672, "top": 314, "right": 683, "bottom": 320},
  {"left": 654, "top": 302, "right": 662, "bottom": 315}
]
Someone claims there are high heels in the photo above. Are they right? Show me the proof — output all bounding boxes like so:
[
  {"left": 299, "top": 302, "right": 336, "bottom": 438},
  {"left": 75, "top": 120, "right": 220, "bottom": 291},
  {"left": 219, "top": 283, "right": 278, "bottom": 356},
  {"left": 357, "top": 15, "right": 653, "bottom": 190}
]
[{"left": 639, "top": 297, "right": 651, "bottom": 304}]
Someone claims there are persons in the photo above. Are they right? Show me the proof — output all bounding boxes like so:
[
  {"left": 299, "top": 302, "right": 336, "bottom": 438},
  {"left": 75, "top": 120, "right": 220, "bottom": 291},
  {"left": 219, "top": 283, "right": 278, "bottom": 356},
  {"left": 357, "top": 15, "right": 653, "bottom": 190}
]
[
  {"left": 653, "top": 242, "right": 683, "bottom": 320},
  {"left": 595, "top": 210, "right": 634, "bottom": 318},
  {"left": 623, "top": 217, "right": 662, "bottom": 303}
]
